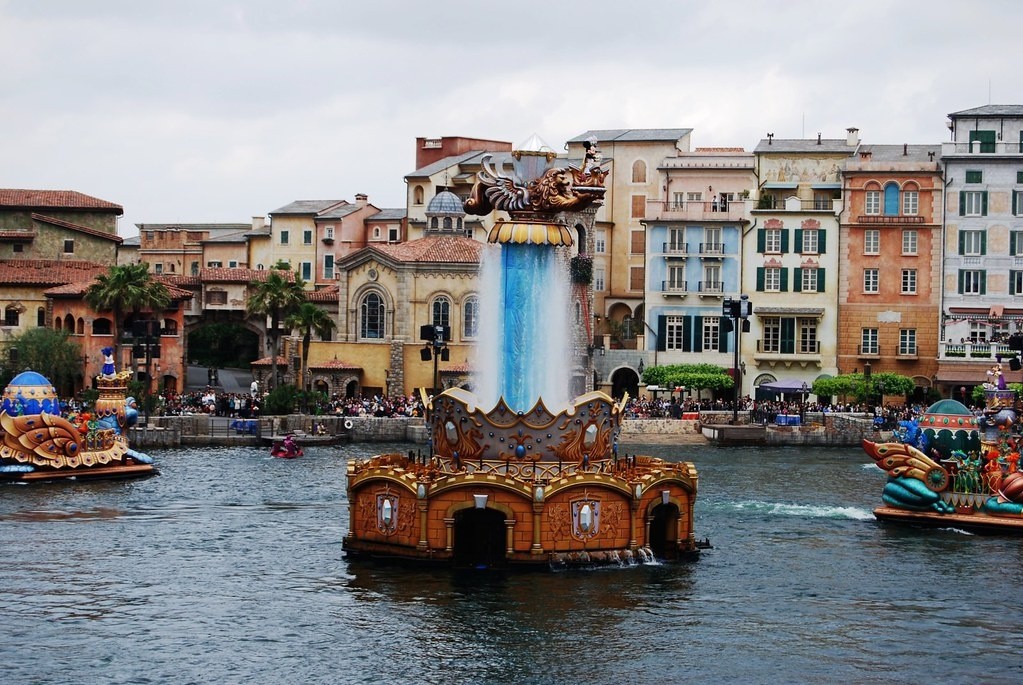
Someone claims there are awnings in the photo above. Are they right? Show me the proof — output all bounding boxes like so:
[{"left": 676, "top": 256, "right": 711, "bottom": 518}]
[
  {"left": 250, "top": 355, "right": 289, "bottom": 364},
  {"left": 309, "top": 358, "right": 362, "bottom": 370},
  {"left": 759, "top": 377, "right": 811, "bottom": 391}
]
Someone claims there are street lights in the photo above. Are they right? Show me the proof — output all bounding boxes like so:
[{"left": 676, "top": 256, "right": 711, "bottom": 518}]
[
  {"left": 719, "top": 294, "right": 752, "bottom": 420},
  {"left": 129, "top": 319, "right": 163, "bottom": 422},
  {"left": 419, "top": 324, "right": 451, "bottom": 394}
]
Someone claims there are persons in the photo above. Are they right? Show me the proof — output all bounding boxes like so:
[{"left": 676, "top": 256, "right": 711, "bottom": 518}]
[
  {"left": 317, "top": 422, "right": 326, "bottom": 436},
  {"left": 614, "top": 394, "right": 930, "bottom": 431},
  {"left": 612, "top": 438, "right": 619, "bottom": 461},
  {"left": 60, "top": 378, "right": 425, "bottom": 417},
  {"left": 712, "top": 195, "right": 727, "bottom": 212},
  {"left": 284, "top": 436, "right": 297, "bottom": 454},
  {"left": 948, "top": 332, "right": 1011, "bottom": 345}
]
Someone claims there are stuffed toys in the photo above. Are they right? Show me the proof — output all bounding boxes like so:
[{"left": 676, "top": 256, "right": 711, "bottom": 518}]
[
  {"left": 581, "top": 141, "right": 598, "bottom": 174},
  {"left": 101, "top": 347, "right": 118, "bottom": 378},
  {"left": 986, "top": 364, "right": 1005, "bottom": 390}
]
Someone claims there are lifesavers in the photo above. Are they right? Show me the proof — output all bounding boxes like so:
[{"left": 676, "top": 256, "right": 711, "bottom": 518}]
[{"left": 344, "top": 420, "right": 354, "bottom": 429}]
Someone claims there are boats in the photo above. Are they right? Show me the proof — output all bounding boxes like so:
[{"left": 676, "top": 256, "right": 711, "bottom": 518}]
[
  {"left": 0, "top": 369, "right": 160, "bottom": 483},
  {"left": 860, "top": 399, "right": 1023, "bottom": 535}
]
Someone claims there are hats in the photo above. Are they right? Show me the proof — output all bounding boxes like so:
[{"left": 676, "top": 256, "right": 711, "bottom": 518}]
[{"left": 256, "top": 380, "right": 259, "bottom": 383}]
